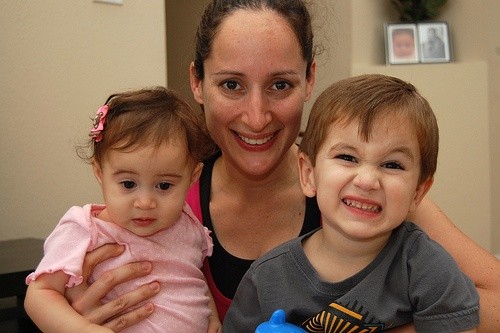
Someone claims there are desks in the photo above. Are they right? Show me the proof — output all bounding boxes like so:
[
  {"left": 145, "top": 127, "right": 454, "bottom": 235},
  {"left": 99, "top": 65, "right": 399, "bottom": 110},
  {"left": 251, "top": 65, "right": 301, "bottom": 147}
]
[{"left": 0, "top": 238, "right": 45, "bottom": 333}]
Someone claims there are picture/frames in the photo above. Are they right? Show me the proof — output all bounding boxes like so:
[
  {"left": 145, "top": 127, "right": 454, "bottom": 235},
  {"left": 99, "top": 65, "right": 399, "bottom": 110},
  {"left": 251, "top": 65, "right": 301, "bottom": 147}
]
[{"left": 383, "top": 21, "right": 454, "bottom": 67}]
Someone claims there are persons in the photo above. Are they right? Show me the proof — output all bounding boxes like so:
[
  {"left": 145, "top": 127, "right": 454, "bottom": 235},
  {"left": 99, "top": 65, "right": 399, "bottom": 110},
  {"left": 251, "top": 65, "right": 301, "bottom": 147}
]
[
  {"left": 392, "top": 28, "right": 415, "bottom": 59},
  {"left": 421, "top": 27, "right": 444, "bottom": 58},
  {"left": 224, "top": 73, "right": 479, "bottom": 333},
  {"left": 63, "top": 0, "right": 499, "bottom": 333},
  {"left": 25, "top": 87, "right": 223, "bottom": 333}
]
